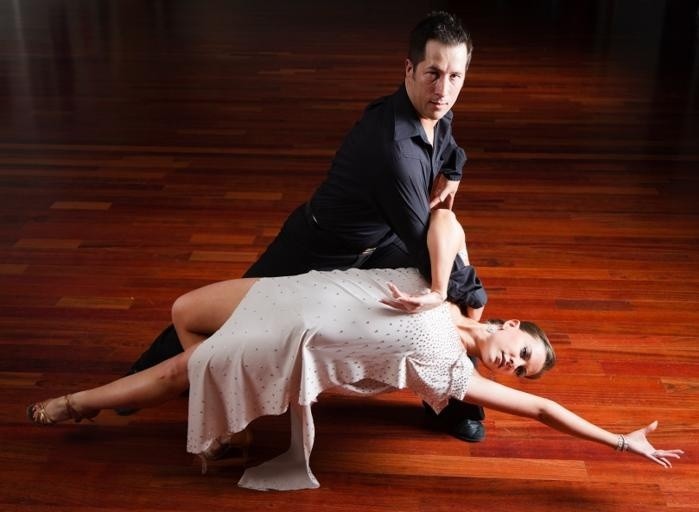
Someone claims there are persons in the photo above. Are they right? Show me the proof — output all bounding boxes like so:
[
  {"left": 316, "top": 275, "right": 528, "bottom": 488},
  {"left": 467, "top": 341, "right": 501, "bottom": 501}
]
[
  {"left": 23, "top": 205, "right": 688, "bottom": 497},
  {"left": 107, "top": 5, "right": 492, "bottom": 448}
]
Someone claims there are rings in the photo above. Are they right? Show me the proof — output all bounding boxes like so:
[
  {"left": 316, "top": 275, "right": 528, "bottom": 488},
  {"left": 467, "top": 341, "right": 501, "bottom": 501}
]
[{"left": 653, "top": 450, "right": 658, "bottom": 458}]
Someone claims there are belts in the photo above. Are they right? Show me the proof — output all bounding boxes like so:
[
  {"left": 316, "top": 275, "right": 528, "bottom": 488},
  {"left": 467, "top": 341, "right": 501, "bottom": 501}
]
[{"left": 304, "top": 199, "right": 331, "bottom": 236}]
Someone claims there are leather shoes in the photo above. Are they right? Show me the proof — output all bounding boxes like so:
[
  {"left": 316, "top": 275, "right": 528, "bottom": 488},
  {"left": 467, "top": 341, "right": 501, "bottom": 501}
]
[
  {"left": 446, "top": 417, "right": 487, "bottom": 442},
  {"left": 115, "top": 366, "right": 147, "bottom": 416}
]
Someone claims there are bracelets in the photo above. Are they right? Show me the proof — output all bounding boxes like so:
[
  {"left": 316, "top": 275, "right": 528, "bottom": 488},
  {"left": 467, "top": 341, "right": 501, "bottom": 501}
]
[
  {"left": 616, "top": 432, "right": 629, "bottom": 454},
  {"left": 432, "top": 285, "right": 447, "bottom": 302}
]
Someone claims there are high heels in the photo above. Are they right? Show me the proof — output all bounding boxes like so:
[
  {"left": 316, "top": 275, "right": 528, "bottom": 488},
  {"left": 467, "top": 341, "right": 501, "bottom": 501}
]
[
  {"left": 203, "top": 428, "right": 253, "bottom": 460},
  {"left": 26, "top": 392, "right": 101, "bottom": 426}
]
